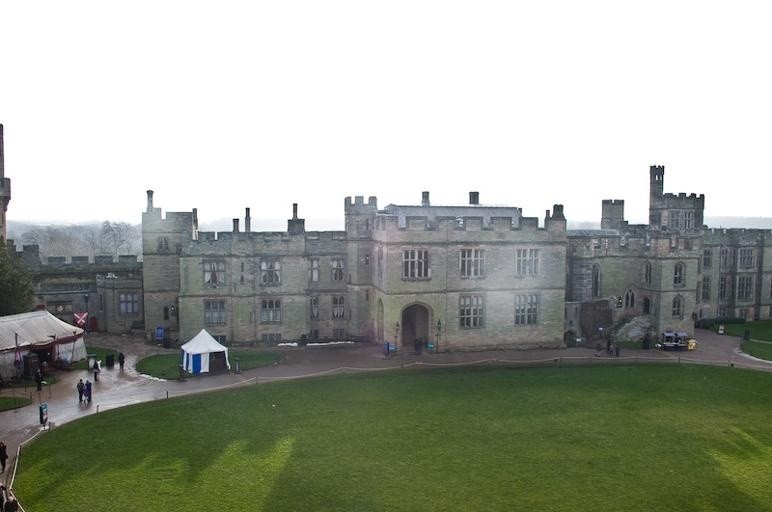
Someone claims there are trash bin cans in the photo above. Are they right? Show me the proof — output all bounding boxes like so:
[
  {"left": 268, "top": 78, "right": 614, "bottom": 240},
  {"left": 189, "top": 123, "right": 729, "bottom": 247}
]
[
  {"left": 87, "top": 355, "right": 96, "bottom": 369},
  {"left": 39, "top": 403, "right": 47, "bottom": 430},
  {"left": 384, "top": 342, "right": 389, "bottom": 354},
  {"left": 687, "top": 337, "right": 696, "bottom": 351},
  {"left": 106, "top": 352, "right": 114, "bottom": 367},
  {"left": 745, "top": 329, "right": 751, "bottom": 341},
  {"left": 235, "top": 360, "right": 240, "bottom": 374}
]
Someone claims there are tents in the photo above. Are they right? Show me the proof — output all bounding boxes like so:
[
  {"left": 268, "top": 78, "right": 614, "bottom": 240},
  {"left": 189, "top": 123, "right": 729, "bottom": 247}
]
[
  {"left": 179, "top": 329, "right": 231, "bottom": 376},
  {"left": 0, "top": 310, "right": 88, "bottom": 384}
]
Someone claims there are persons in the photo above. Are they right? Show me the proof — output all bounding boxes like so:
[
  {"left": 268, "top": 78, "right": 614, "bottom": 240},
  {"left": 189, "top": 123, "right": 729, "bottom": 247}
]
[{"left": 0, "top": 352, "right": 124, "bottom": 512}]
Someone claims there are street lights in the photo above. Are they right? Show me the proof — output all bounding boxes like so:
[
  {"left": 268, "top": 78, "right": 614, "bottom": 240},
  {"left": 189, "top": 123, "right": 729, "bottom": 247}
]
[
  {"left": 435, "top": 320, "right": 442, "bottom": 354},
  {"left": 598, "top": 327, "right": 604, "bottom": 339},
  {"left": 393, "top": 322, "right": 401, "bottom": 351}
]
